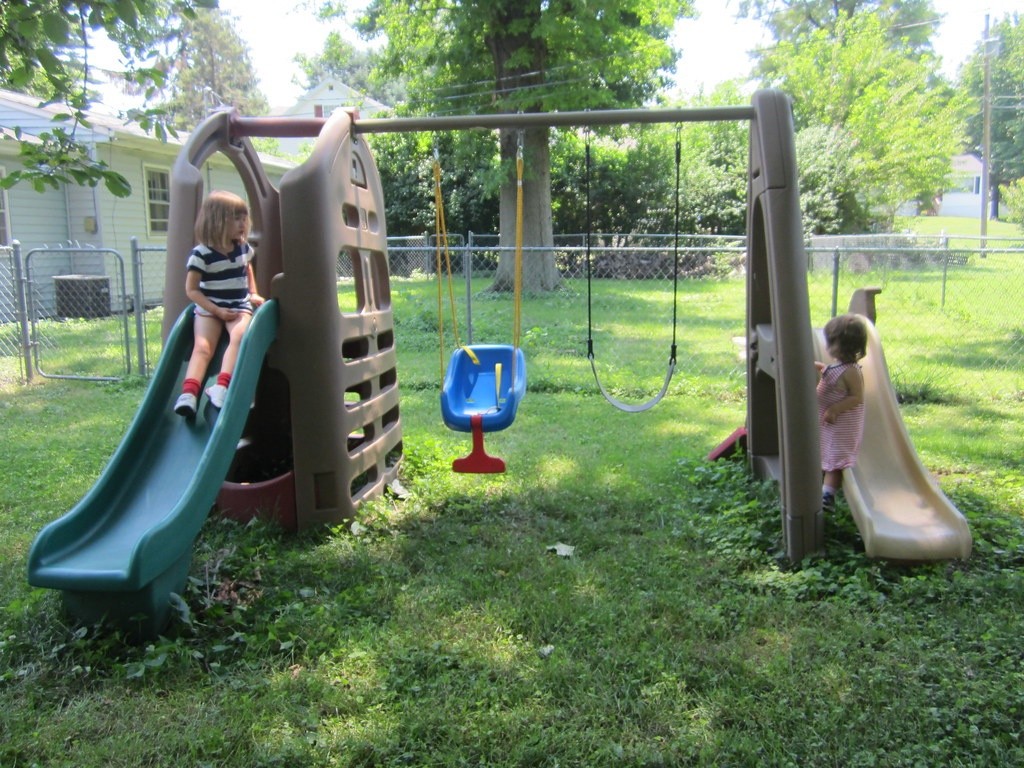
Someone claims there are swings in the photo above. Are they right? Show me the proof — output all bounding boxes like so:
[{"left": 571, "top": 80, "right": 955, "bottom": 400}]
[
  {"left": 582, "top": 120, "right": 683, "bottom": 413},
  {"left": 431, "top": 128, "right": 527, "bottom": 432}
]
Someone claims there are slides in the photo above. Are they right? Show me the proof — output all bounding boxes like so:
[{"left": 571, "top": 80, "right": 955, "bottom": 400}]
[
  {"left": 26, "top": 297, "right": 278, "bottom": 595},
  {"left": 807, "top": 316, "right": 974, "bottom": 562}
]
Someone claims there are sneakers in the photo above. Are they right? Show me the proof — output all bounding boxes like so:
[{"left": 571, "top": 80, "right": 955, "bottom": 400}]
[
  {"left": 205, "top": 384, "right": 227, "bottom": 410},
  {"left": 174, "top": 392, "right": 197, "bottom": 416}
]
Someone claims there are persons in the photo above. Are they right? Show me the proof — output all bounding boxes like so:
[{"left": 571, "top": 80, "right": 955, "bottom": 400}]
[
  {"left": 814, "top": 315, "right": 867, "bottom": 513},
  {"left": 173, "top": 191, "right": 265, "bottom": 418}
]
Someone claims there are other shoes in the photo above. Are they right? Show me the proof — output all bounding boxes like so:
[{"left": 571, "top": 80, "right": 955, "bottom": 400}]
[{"left": 822, "top": 491, "right": 836, "bottom": 513}]
